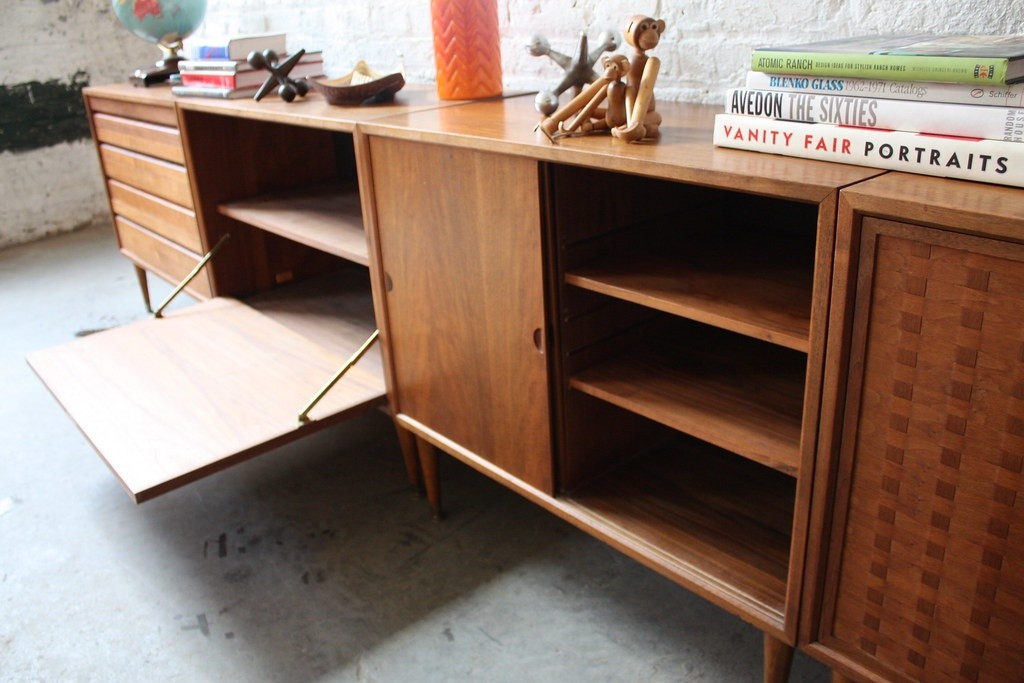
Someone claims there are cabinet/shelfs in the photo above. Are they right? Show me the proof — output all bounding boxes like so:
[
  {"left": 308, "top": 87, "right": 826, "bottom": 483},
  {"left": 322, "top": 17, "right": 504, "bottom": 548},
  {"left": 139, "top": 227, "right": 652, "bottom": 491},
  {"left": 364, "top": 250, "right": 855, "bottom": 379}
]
[{"left": 22, "top": 81, "right": 1022, "bottom": 681}]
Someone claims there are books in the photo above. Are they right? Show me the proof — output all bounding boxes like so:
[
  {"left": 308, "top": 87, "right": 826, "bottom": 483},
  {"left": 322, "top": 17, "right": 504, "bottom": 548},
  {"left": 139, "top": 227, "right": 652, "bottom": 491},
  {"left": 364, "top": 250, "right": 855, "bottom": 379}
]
[
  {"left": 170, "top": 34, "right": 329, "bottom": 99},
  {"left": 713, "top": 33, "right": 1024, "bottom": 188}
]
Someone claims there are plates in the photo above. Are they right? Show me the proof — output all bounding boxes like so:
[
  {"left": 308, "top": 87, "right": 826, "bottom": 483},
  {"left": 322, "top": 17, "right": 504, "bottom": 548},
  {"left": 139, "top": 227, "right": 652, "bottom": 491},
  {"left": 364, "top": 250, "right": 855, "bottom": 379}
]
[{"left": 303, "top": 59, "right": 405, "bottom": 105}]
